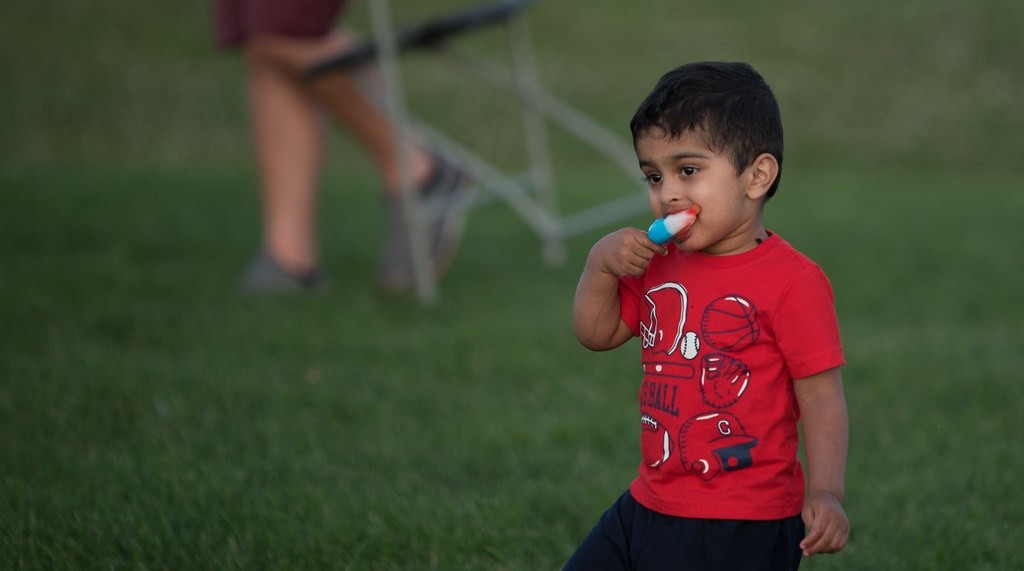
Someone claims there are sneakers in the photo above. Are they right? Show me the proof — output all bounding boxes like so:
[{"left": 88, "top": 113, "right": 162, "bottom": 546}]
[
  {"left": 376, "top": 153, "right": 476, "bottom": 298},
  {"left": 229, "top": 245, "right": 331, "bottom": 303}
]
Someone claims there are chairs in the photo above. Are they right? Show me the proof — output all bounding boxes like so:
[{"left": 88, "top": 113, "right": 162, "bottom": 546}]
[{"left": 300, "top": 1, "right": 652, "bottom": 311}]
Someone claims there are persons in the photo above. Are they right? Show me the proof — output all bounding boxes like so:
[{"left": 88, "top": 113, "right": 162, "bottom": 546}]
[
  {"left": 559, "top": 61, "right": 851, "bottom": 571},
  {"left": 205, "top": 0, "right": 471, "bottom": 295}
]
